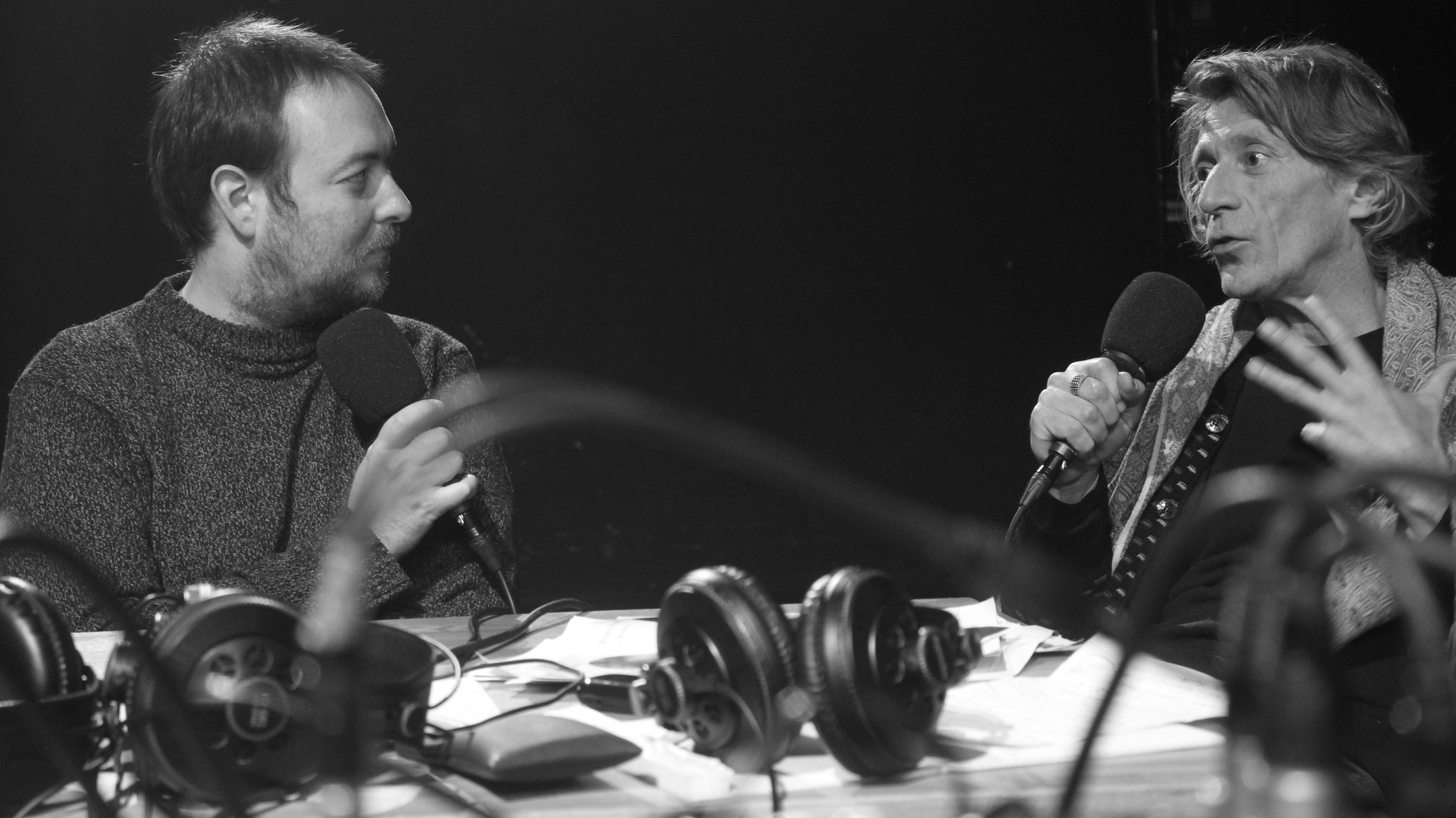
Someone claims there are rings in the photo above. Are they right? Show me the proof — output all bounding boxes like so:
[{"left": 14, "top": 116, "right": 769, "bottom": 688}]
[{"left": 1070, "top": 374, "right": 1087, "bottom": 396}]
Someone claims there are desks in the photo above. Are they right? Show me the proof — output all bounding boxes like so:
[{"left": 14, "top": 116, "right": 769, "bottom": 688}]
[{"left": 27, "top": 596, "right": 1389, "bottom": 818}]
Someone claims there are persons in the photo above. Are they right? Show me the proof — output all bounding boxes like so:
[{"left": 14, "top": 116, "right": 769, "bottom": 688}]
[
  {"left": 0, "top": 11, "right": 519, "bottom": 635},
  {"left": 1007, "top": 41, "right": 1456, "bottom": 818}
]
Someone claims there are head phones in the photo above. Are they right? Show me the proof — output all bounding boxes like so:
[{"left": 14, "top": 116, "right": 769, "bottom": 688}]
[
  {"left": 650, "top": 563, "right": 972, "bottom": 782},
  {"left": 0, "top": 574, "right": 436, "bottom": 814}
]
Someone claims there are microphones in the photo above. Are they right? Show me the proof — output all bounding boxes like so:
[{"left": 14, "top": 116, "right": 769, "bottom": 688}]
[{"left": 319, "top": 309, "right": 505, "bottom": 573}]
